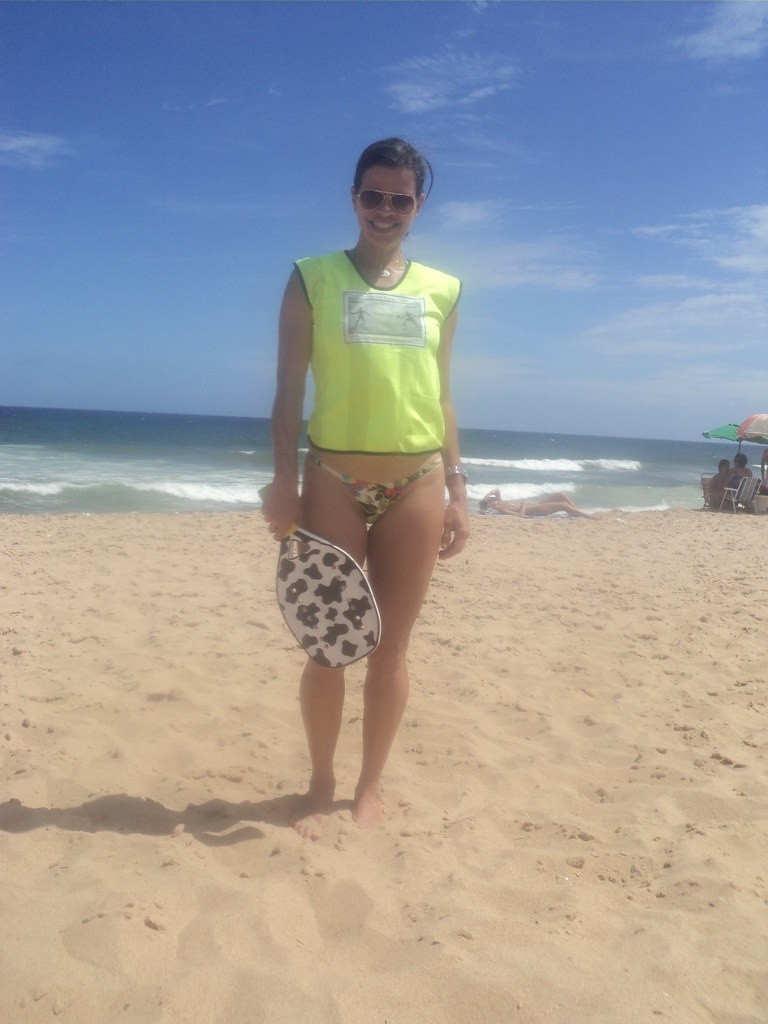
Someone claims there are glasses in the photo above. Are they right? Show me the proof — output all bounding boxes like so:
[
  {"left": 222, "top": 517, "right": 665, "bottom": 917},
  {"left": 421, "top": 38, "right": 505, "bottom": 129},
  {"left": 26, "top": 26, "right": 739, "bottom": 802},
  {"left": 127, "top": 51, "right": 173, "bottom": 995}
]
[{"left": 357, "top": 189, "right": 417, "bottom": 216}]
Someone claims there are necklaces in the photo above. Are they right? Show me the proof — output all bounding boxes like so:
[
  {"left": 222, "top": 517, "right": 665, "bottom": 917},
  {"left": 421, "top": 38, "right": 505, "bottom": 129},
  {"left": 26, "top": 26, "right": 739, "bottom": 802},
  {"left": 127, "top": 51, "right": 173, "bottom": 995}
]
[{"left": 353, "top": 244, "right": 405, "bottom": 278}]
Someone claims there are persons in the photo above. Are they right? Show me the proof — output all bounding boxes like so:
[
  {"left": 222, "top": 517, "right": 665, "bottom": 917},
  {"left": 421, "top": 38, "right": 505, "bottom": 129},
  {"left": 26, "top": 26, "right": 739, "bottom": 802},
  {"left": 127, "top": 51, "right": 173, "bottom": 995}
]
[
  {"left": 479, "top": 489, "right": 603, "bottom": 520},
  {"left": 708, "top": 459, "right": 738, "bottom": 512},
  {"left": 261, "top": 136, "right": 471, "bottom": 825},
  {"left": 715, "top": 453, "right": 753, "bottom": 510}
]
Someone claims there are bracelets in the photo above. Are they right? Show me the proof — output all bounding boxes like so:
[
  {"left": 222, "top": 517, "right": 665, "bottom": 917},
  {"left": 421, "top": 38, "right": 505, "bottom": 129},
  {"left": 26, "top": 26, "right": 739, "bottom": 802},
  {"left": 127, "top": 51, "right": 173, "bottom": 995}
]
[{"left": 443, "top": 464, "right": 469, "bottom": 487}]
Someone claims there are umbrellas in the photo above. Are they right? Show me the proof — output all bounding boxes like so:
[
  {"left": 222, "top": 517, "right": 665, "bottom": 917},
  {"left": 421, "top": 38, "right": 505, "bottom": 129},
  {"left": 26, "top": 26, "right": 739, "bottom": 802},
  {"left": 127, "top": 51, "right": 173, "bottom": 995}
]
[
  {"left": 702, "top": 423, "right": 744, "bottom": 454},
  {"left": 736, "top": 413, "right": 768, "bottom": 445}
]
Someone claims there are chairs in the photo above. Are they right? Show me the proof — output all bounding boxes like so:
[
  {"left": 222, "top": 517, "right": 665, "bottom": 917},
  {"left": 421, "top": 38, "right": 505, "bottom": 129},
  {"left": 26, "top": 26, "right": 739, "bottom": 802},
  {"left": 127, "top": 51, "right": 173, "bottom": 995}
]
[
  {"left": 719, "top": 476, "right": 762, "bottom": 517},
  {"left": 700, "top": 472, "right": 720, "bottom": 509}
]
